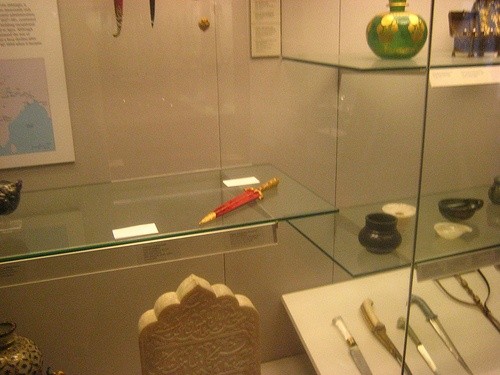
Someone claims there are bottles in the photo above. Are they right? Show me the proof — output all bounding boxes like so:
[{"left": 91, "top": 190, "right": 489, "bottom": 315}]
[
  {"left": 358, "top": 212, "right": 403, "bottom": 255},
  {"left": 365, "top": 0, "right": 428, "bottom": 60},
  {"left": 0, "top": 321, "right": 43, "bottom": 375},
  {"left": 488, "top": 175, "right": 500, "bottom": 204}
]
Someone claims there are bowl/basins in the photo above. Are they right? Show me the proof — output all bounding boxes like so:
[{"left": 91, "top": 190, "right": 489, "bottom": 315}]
[{"left": 438, "top": 197, "right": 484, "bottom": 221}]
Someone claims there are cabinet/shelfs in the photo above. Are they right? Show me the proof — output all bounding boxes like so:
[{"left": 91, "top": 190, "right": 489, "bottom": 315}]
[{"left": 286, "top": 53, "right": 500, "bottom": 277}]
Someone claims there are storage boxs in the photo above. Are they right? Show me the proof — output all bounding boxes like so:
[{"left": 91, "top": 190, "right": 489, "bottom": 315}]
[{"left": 449, "top": 11, "right": 500, "bottom": 57}]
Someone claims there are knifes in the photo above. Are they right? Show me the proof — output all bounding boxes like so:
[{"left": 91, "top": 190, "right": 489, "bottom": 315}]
[
  {"left": 333, "top": 317, "right": 372, "bottom": 375},
  {"left": 363, "top": 298, "right": 413, "bottom": 375},
  {"left": 408, "top": 293, "right": 473, "bottom": 375},
  {"left": 400, "top": 317, "right": 439, "bottom": 375}
]
